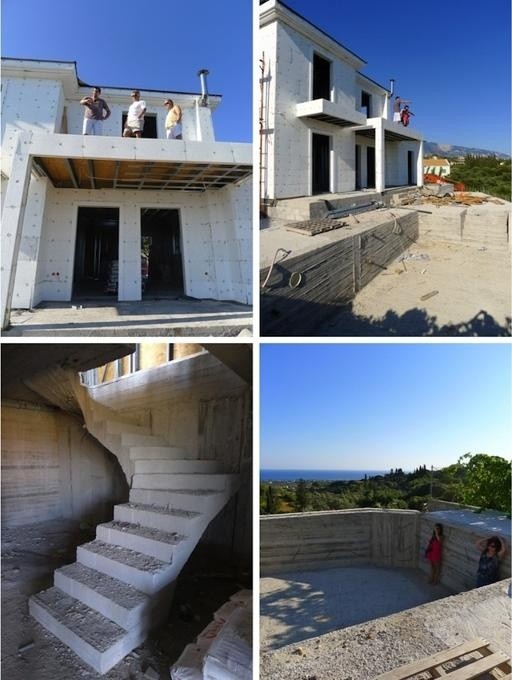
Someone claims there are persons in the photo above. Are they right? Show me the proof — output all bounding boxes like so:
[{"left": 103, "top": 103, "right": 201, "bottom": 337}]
[
  {"left": 474, "top": 535, "right": 506, "bottom": 588},
  {"left": 423, "top": 523, "right": 443, "bottom": 587},
  {"left": 399, "top": 102, "right": 415, "bottom": 127},
  {"left": 161, "top": 97, "right": 182, "bottom": 140},
  {"left": 393, "top": 96, "right": 413, "bottom": 124},
  {"left": 124, "top": 89, "right": 147, "bottom": 138},
  {"left": 80, "top": 85, "right": 111, "bottom": 135}
]
